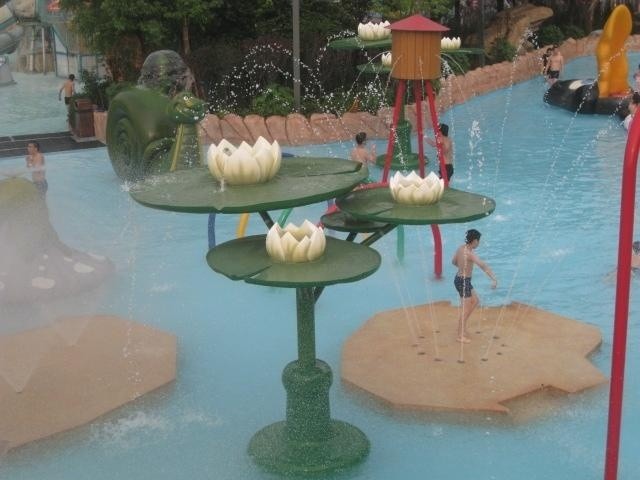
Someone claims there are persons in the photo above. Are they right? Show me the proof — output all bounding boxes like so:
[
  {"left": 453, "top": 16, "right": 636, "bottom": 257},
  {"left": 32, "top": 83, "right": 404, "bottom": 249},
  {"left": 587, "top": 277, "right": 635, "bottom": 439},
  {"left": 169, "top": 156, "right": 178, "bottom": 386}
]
[
  {"left": 58, "top": 74, "right": 76, "bottom": 113},
  {"left": 423, "top": 124, "right": 454, "bottom": 187},
  {"left": 634, "top": 65, "right": 640, "bottom": 74},
  {"left": 452, "top": 229, "right": 499, "bottom": 343},
  {"left": 26, "top": 141, "right": 48, "bottom": 203},
  {"left": 628, "top": 94, "right": 640, "bottom": 114},
  {"left": 539, "top": 42, "right": 567, "bottom": 84},
  {"left": 351, "top": 132, "right": 376, "bottom": 171},
  {"left": 631, "top": 74, "right": 640, "bottom": 95},
  {"left": 601, "top": 241, "right": 639, "bottom": 288}
]
